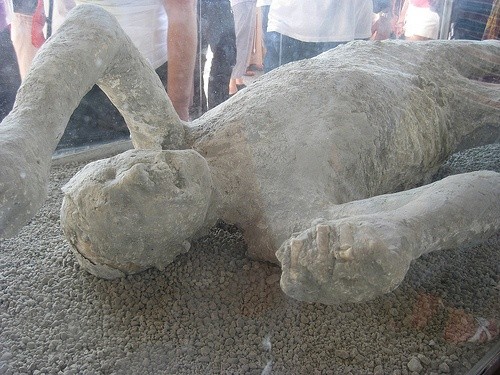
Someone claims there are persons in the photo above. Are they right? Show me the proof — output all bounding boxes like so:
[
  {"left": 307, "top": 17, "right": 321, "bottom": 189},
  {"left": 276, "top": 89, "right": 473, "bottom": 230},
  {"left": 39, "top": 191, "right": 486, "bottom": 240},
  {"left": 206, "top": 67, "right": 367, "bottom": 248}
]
[
  {"left": 435, "top": 0, "right": 499, "bottom": 39},
  {"left": 10, "top": 0, "right": 47, "bottom": 84},
  {"left": 187, "top": 0, "right": 237, "bottom": 124},
  {"left": 267, "top": 1, "right": 395, "bottom": 74},
  {"left": 228, "top": 1, "right": 258, "bottom": 97},
  {"left": 50, "top": 0, "right": 76, "bottom": 151},
  {"left": 0, "top": 0, "right": 23, "bottom": 124},
  {"left": 2, "top": 5, "right": 500, "bottom": 303},
  {"left": 243, "top": 0, "right": 264, "bottom": 77},
  {"left": 74, "top": 0, "right": 200, "bottom": 153},
  {"left": 403, "top": 0, "right": 441, "bottom": 42}
]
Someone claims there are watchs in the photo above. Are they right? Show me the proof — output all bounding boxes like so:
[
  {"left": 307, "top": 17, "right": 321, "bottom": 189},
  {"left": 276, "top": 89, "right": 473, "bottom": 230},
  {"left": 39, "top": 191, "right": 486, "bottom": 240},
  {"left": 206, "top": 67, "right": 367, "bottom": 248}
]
[{"left": 379, "top": 11, "right": 393, "bottom": 20}]
